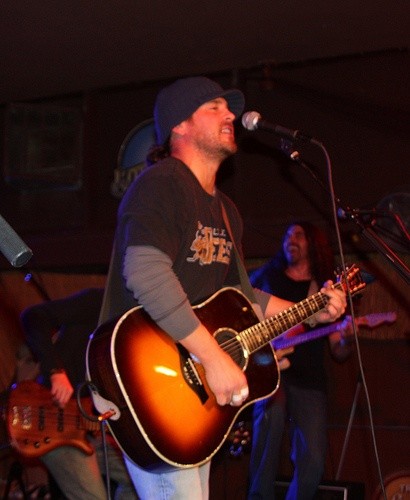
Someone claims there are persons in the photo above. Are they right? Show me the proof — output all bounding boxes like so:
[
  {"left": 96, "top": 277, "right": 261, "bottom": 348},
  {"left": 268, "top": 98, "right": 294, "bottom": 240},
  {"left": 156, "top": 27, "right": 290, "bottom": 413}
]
[
  {"left": 248, "top": 218, "right": 336, "bottom": 500},
  {"left": 95, "top": 75, "right": 348, "bottom": 500},
  {"left": 15, "top": 284, "right": 141, "bottom": 500}
]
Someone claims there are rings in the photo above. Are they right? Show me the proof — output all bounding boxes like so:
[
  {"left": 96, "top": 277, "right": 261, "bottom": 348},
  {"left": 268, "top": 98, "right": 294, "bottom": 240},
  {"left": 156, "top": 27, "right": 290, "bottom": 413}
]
[
  {"left": 241, "top": 387, "right": 249, "bottom": 396},
  {"left": 232, "top": 395, "right": 242, "bottom": 402}
]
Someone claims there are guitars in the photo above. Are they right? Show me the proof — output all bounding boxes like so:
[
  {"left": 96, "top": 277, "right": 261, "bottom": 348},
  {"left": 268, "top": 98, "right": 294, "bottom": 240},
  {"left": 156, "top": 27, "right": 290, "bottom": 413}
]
[
  {"left": 82, "top": 263, "right": 373, "bottom": 478},
  {"left": 271, "top": 311, "right": 397, "bottom": 371},
  {"left": 0, "top": 378, "right": 253, "bottom": 460}
]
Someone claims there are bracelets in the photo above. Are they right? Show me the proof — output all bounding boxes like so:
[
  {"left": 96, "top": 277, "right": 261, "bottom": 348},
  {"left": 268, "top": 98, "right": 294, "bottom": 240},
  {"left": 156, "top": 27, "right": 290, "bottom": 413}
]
[{"left": 309, "top": 316, "right": 321, "bottom": 328}]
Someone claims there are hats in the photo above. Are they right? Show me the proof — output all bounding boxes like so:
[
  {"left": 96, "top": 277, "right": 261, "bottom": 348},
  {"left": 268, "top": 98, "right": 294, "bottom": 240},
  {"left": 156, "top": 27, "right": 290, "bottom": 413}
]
[{"left": 153, "top": 77, "right": 246, "bottom": 145}]
